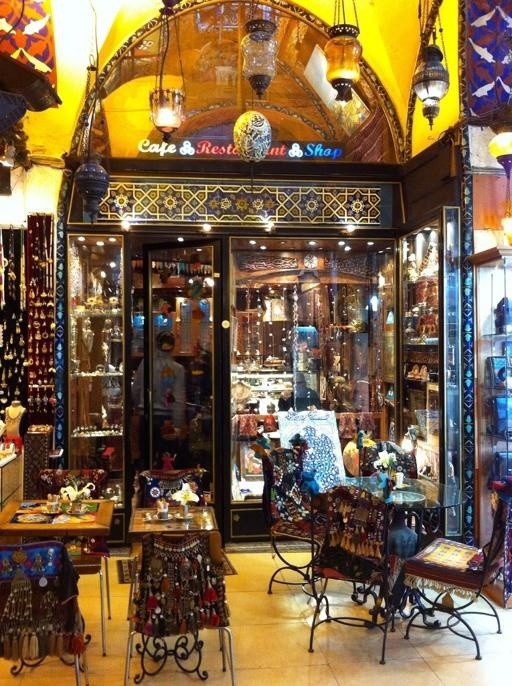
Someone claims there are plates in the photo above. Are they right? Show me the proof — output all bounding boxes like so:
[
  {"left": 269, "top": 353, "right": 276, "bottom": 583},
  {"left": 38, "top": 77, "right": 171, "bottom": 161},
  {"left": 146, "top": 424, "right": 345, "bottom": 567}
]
[
  {"left": 152, "top": 514, "right": 173, "bottom": 521},
  {"left": 174, "top": 512, "right": 194, "bottom": 520}
]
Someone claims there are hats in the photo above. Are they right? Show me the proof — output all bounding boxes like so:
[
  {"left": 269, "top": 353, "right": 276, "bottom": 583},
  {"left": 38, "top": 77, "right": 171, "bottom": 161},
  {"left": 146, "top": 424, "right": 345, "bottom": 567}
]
[{"left": 156, "top": 331, "right": 174, "bottom": 351}]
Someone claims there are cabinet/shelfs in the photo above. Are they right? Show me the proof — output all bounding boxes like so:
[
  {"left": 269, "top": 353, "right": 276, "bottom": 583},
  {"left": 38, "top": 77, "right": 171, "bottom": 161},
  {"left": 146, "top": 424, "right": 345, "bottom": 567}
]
[
  {"left": 400, "top": 207, "right": 462, "bottom": 543},
  {"left": 68, "top": 234, "right": 125, "bottom": 547},
  {"left": 469, "top": 246, "right": 512, "bottom": 608}
]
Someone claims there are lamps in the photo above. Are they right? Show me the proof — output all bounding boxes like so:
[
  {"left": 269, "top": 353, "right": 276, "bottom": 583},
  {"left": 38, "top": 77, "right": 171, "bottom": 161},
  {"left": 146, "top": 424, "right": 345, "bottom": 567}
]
[{"left": 478, "top": 104, "right": 512, "bottom": 247}]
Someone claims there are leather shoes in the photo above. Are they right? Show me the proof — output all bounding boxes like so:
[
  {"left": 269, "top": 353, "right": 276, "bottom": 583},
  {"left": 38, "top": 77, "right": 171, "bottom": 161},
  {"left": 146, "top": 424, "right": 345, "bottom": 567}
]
[{"left": 407, "top": 364, "right": 428, "bottom": 379}]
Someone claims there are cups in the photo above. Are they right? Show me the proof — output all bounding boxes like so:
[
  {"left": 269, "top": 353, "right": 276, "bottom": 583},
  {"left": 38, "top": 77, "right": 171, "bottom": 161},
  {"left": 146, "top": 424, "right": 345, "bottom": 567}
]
[
  {"left": 156, "top": 508, "right": 169, "bottom": 519},
  {"left": 179, "top": 504, "right": 188, "bottom": 517},
  {"left": 70, "top": 500, "right": 81, "bottom": 513}
]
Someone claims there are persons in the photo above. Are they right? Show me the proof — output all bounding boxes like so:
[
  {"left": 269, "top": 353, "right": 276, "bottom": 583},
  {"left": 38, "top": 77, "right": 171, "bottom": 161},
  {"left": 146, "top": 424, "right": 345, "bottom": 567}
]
[
  {"left": 278, "top": 372, "right": 359, "bottom": 449},
  {"left": 130, "top": 331, "right": 187, "bottom": 469}
]
